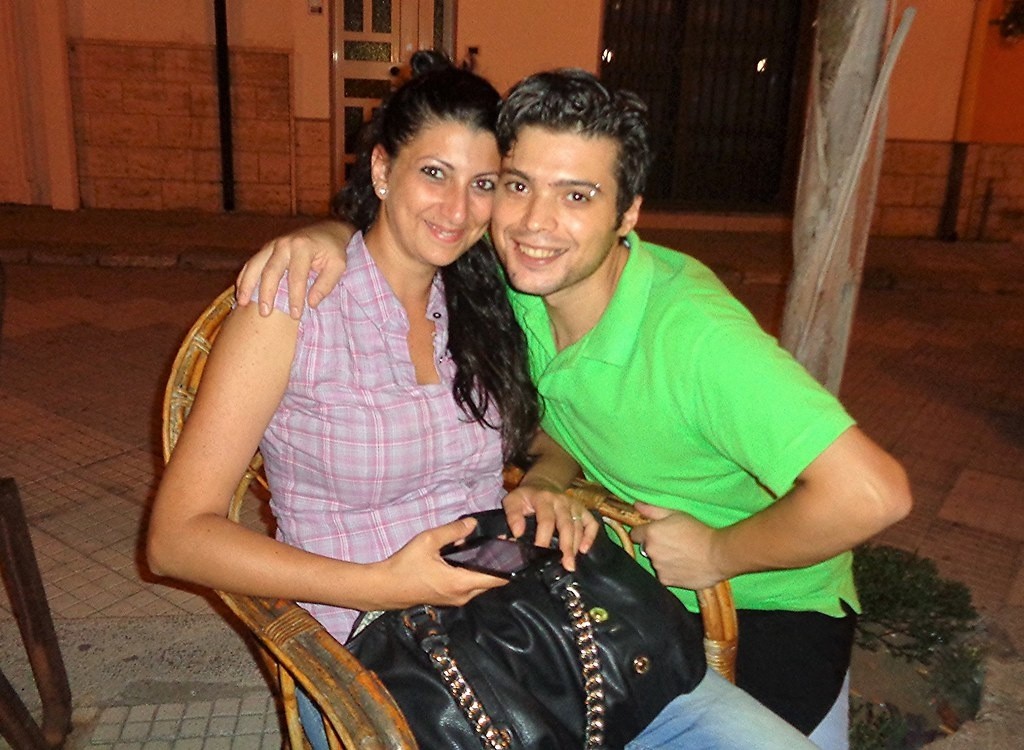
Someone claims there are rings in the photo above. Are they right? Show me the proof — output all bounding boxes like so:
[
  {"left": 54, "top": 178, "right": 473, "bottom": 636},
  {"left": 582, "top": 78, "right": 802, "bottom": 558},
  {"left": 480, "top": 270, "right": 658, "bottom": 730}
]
[
  {"left": 639, "top": 543, "right": 647, "bottom": 557},
  {"left": 572, "top": 516, "right": 582, "bottom": 520}
]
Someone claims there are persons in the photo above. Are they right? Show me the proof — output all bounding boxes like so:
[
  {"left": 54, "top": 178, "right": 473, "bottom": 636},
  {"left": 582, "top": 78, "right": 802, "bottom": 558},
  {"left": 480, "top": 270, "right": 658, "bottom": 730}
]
[
  {"left": 146, "top": 49, "right": 818, "bottom": 750},
  {"left": 235, "top": 66, "right": 914, "bottom": 750}
]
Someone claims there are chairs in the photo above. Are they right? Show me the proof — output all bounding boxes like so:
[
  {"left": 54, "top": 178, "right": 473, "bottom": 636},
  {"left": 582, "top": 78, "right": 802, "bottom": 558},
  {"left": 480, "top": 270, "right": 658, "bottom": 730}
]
[{"left": 159, "top": 286, "right": 738, "bottom": 750}]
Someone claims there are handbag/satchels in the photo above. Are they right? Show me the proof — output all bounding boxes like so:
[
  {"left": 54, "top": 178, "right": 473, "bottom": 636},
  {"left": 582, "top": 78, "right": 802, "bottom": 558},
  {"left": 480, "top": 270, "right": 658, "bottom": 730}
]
[{"left": 337, "top": 506, "right": 709, "bottom": 750}]
